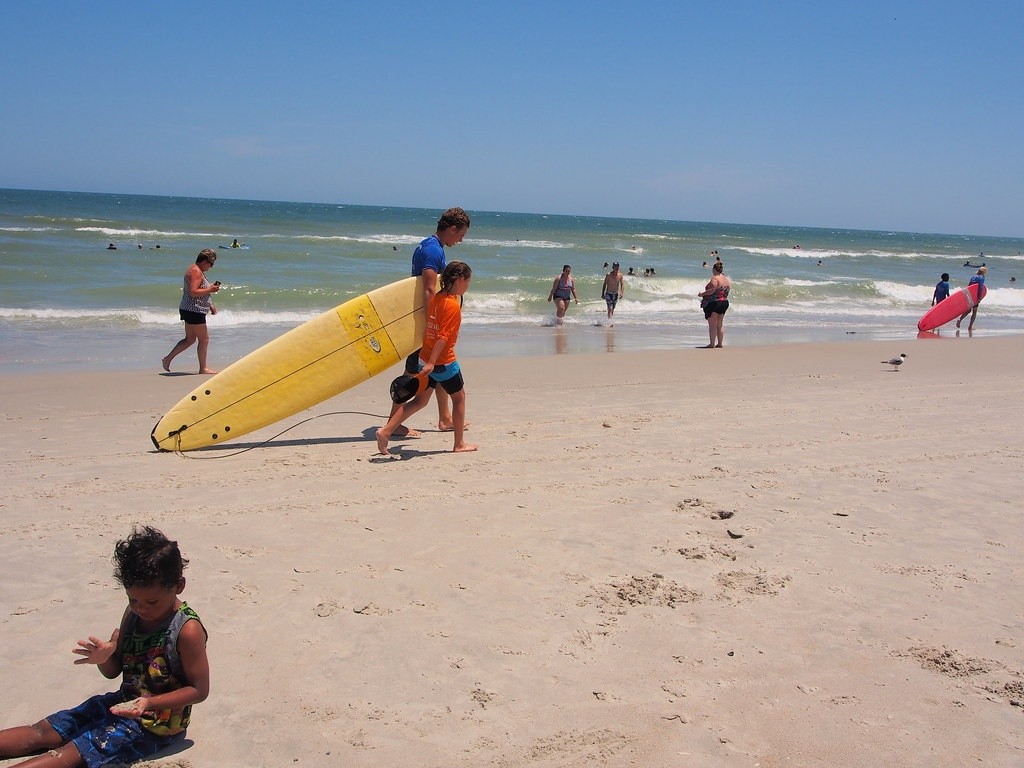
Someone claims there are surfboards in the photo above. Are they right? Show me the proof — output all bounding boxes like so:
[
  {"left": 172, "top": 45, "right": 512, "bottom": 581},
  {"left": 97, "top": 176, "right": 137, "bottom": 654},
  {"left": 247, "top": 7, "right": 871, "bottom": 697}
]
[
  {"left": 918, "top": 282, "right": 988, "bottom": 331},
  {"left": 150, "top": 272, "right": 445, "bottom": 454}
]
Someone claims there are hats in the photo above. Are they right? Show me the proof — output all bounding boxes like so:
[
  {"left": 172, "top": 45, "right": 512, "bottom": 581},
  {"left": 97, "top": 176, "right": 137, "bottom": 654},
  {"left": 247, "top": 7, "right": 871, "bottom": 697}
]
[{"left": 389, "top": 373, "right": 429, "bottom": 404}]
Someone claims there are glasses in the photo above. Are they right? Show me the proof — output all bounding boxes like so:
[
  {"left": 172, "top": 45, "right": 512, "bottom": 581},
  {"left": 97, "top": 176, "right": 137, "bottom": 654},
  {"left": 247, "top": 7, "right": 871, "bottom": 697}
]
[{"left": 209, "top": 261, "right": 215, "bottom": 267}]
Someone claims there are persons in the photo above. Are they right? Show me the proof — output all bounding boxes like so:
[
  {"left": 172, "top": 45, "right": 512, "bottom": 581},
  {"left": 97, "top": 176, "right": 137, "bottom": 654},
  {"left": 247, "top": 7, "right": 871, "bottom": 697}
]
[
  {"left": 388, "top": 207, "right": 471, "bottom": 436},
  {"left": 956, "top": 267, "right": 986, "bottom": 330},
  {"left": 698, "top": 262, "right": 730, "bottom": 348},
  {"left": 374, "top": 261, "right": 479, "bottom": 455},
  {"left": 602, "top": 244, "right": 822, "bottom": 277},
  {"left": 161, "top": 248, "right": 220, "bottom": 375},
  {"left": 932, "top": 273, "right": 949, "bottom": 306},
  {"left": 547, "top": 265, "right": 578, "bottom": 325},
  {"left": 602, "top": 262, "right": 624, "bottom": 327},
  {"left": 0, "top": 525, "right": 210, "bottom": 768},
  {"left": 231, "top": 239, "right": 241, "bottom": 248},
  {"left": 964, "top": 253, "right": 1016, "bottom": 281}
]
